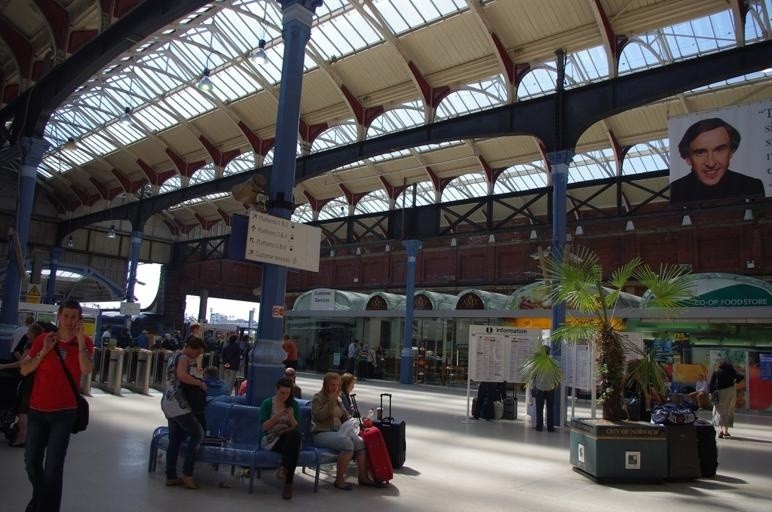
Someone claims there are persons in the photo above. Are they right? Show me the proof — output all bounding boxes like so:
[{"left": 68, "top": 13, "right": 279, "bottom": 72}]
[
  {"left": 671, "top": 119, "right": 765, "bottom": 203},
  {"left": 689, "top": 374, "right": 709, "bottom": 410},
  {"left": 474, "top": 382, "right": 507, "bottom": 421},
  {"left": 709, "top": 359, "right": 744, "bottom": 438},
  {"left": 1, "top": 298, "right": 385, "bottom": 512},
  {"left": 530, "top": 345, "right": 560, "bottom": 432}
]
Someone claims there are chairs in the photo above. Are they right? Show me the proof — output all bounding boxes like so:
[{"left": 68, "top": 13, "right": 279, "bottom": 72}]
[{"left": 149, "top": 395, "right": 367, "bottom": 494}]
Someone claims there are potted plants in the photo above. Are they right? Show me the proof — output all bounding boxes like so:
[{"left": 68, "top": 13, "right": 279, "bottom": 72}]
[{"left": 512, "top": 244, "right": 698, "bottom": 484}]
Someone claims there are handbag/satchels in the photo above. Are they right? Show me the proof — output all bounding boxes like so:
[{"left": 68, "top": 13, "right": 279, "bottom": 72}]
[
  {"left": 180, "top": 375, "right": 207, "bottom": 412},
  {"left": 73, "top": 395, "right": 89, "bottom": 434},
  {"left": 651, "top": 402, "right": 696, "bottom": 424},
  {"left": 338, "top": 418, "right": 360, "bottom": 438},
  {"left": 711, "top": 392, "right": 719, "bottom": 405},
  {"left": 260, "top": 433, "right": 280, "bottom": 451}
]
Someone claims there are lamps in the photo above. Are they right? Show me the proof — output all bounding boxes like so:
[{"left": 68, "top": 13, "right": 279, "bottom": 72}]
[
  {"left": 198, "top": 16, "right": 215, "bottom": 92},
  {"left": 120, "top": 64, "right": 135, "bottom": 125},
  {"left": 352, "top": 276, "right": 360, "bottom": 284},
  {"left": 66, "top": 235, "right": 76, "bottom": 249},
  {"left": 744, "top": 256, "right": 761, "bottom": 270},
  {"left": 251, "top": 2, "right": 269, "bottom": 66},
  {"left": 107, "top": 225, "right": 116, "bottom": 239},
  {"left": 65, "top": 102, "right": 78, "bottom": 153}
]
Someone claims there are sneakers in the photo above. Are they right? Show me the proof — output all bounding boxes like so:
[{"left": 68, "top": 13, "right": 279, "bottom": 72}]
[
  {"left": 277, "top": 465, "right": 288, "bottom": 480},
  {"left": 333, "top": 481, "right": 353, "bottom": 490},
  {"left": 181, "top": 475, "right": 197, "bottom": 489},
  {"left": 165, "top": 478, "right": 185, "bottom": 486},
  {"left": 8, "top": 439, "right": 25, "bottom": 447},
  {"left": 358, "top": 477, "right": 375, "bottom": 485},
  {"left": 719, "top": 431, "right": 730, "bottom": 438},
  {"left": 283, "top": 483, "right": 293, "bottom": 498}
]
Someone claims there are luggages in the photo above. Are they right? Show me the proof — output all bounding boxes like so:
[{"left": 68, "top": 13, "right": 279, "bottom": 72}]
[
  {"left": 669, "top": 420, "right": 718, "bottom": 481},
  {"left": 472, "top": 382, "right": 518, "bottom": 420},
  {"left": 234, "top": 375, "right": 245, "bottom": 397},
  {"left": 352, "top": 393, "right": 393, "bottom": 484},
  {"left": 372, "top": 392, "right": 406, "bottom": 469}
]
